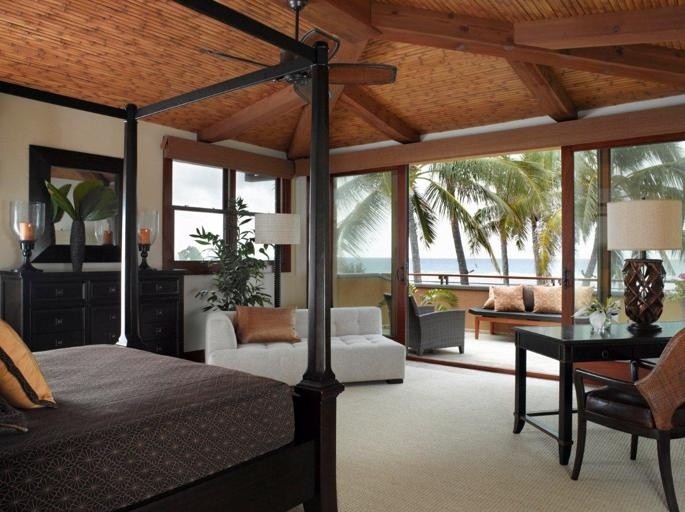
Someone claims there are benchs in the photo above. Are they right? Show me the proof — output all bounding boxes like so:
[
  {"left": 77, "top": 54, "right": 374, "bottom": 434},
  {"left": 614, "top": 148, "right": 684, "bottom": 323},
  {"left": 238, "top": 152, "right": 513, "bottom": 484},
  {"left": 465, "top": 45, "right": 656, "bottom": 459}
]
[{"left": 468, "top": 306, "right": 595, "bottom": 342}]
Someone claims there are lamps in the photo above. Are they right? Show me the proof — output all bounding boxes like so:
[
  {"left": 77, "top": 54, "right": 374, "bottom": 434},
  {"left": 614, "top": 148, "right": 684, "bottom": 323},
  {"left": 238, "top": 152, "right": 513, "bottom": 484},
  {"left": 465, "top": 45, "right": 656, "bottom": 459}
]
[
  {"left": 604, "top": 197, "right": 683, "bottom": 334},
  {"left": 251, "top": 210, "right": 304, "bottom": 308}
]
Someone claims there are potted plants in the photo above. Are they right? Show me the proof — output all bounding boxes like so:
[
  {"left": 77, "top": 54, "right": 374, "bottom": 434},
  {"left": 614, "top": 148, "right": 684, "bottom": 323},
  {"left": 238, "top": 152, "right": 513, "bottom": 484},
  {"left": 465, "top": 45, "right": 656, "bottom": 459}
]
[
  {"left": 45, "top": 177, "right": 123, "bottom": 275},
  {"left": 571, "top": 296, "right": 623, "bottom": 331}
]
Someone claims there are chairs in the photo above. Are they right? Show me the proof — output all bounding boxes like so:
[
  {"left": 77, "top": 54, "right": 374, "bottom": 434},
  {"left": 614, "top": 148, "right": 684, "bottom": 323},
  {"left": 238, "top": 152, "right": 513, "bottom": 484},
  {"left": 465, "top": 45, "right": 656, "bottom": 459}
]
[
  {"left": 569, "top": 325, "right": 683, "bottom": 512},
  {"left": 233, "top": 304, "right": 303, "bottom": 345}
]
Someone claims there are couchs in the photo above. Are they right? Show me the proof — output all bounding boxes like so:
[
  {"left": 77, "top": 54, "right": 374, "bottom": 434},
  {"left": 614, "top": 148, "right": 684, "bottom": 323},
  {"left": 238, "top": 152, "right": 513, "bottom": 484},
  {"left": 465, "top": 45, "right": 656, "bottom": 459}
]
[
  {"left": 384, "top": 292, "right": 468, "bottom": 356},
  {"left": 201, "top": 305, "right": 406, "bottom": 390}
]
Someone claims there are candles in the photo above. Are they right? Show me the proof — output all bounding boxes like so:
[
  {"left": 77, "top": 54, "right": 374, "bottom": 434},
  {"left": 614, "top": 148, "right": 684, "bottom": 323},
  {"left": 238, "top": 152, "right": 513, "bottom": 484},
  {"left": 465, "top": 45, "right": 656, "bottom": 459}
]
[
  {"left": 103, "top": 230, "right": 113, "bottom": 244},
  {"left": 136, "top": 227, "right": 152, "bottom": 245},
  {"left": 19, "top": 220, "right": 36, "bottom": 242}
]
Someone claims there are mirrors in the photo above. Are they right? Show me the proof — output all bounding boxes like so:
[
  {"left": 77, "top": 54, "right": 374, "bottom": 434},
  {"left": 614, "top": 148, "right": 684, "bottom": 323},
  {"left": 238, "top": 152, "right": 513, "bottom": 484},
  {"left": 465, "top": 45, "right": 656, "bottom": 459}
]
[{"left": 26, "top": 143, "right": 125, "bottom": 264}]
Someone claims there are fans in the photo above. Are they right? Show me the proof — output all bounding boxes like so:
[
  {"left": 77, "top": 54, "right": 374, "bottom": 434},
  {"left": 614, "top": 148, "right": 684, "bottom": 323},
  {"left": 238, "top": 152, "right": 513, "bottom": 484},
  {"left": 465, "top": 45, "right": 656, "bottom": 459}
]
[{"left": 197, "top": 0, "right": 399, "bottom": 109}]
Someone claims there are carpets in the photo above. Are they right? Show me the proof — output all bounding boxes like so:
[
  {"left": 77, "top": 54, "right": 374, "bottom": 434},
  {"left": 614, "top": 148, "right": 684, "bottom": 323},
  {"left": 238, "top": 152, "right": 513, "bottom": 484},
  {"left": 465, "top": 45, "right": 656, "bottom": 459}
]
[{"left": 335, "top": 359, "right": 683, "bottom": 512}]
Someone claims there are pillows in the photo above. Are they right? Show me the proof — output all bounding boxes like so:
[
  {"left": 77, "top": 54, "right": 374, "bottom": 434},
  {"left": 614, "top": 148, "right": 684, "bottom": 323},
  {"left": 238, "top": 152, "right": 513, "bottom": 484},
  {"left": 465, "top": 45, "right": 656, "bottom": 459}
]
[
  {"left": 482, "top": 282, "right": 596, "bottom": 315},
  {"left": 0, "top": 394, "right": 29, "bottom": 435},
  {"left": 0, "top": 318, "right": 61, "bottom": 410}
]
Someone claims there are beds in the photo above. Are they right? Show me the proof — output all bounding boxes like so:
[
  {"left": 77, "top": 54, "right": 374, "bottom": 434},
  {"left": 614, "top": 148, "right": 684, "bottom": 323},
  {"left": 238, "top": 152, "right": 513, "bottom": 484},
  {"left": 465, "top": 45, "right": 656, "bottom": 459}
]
[{"left": 0, "top": 0, "right": 345, "bottom": 512}]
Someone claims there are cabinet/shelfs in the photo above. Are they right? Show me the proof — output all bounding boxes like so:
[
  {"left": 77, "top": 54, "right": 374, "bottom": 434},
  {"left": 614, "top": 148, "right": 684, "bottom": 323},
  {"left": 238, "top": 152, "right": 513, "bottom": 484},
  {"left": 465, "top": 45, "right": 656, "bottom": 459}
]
[{"left": 0, "top": 269, "right": 191, "bottom": 364}]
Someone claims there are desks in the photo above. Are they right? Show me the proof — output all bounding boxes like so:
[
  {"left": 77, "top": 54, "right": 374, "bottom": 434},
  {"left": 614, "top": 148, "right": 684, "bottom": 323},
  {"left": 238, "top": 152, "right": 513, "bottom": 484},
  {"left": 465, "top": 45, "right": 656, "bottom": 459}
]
[{"left": 509, "top": 319, "right": 685, "bottom": 469}]
